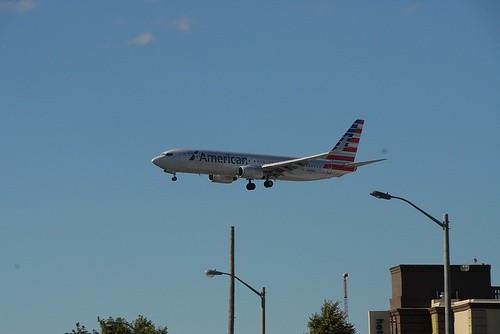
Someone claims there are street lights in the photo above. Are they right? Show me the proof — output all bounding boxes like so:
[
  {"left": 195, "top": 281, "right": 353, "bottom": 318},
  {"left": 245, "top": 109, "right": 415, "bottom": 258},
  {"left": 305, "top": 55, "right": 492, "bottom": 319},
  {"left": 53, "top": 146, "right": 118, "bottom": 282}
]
[
  {"left": 370, "top": 190, "right": 451, "bottom": 333},
  {"left": 205, "top": 269, "right": 265, "bottom": 334}
]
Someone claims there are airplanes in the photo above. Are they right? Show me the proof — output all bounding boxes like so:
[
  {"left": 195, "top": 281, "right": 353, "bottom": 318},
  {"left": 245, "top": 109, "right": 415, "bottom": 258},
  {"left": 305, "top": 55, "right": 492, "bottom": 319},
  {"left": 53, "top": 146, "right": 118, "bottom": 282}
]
[{"left": 152, "top": 119, "right": 386, "bottom": 191}]
